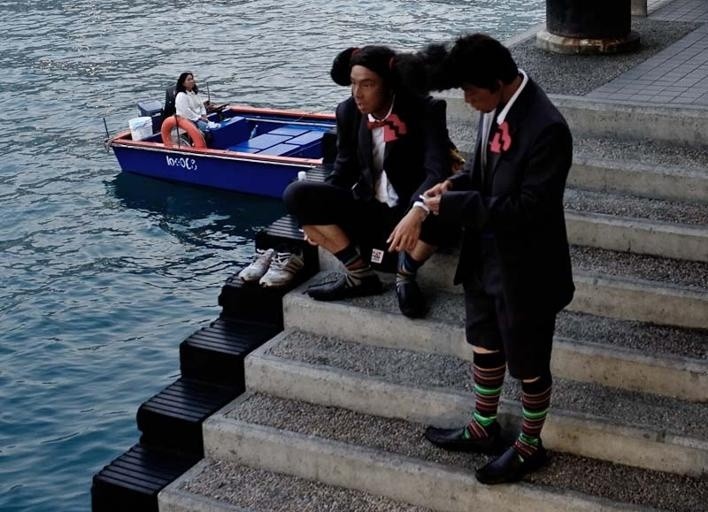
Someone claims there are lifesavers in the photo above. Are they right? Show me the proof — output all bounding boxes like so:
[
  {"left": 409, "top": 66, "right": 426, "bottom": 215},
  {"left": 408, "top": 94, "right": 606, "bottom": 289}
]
[{"left": 161, "top": 117, "right": 206, "bottom": 148}]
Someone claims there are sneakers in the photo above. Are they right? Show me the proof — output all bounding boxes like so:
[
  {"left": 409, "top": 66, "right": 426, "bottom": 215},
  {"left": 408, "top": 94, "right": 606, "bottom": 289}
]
[
  {"left": 206, "top": 121, "right": 223, "bottom": 131},
  {"left": 258, "top": 245, "right": 306, "bottom": 289},
  {"left": 238, "top": 245, "right": 283, "bottom": 284}
]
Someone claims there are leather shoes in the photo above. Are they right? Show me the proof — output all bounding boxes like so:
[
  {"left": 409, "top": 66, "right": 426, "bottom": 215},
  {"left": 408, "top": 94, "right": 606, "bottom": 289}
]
[
  {"left": 307, "top": 275, "right": 383, "bottom": 302},
  {"left": 423, "top": 424, "right": 507, "bottom": 458},
  {"left": 474, "top": 437, "right": 550, "bottom": 485},
  {"left": 392, "top": 278, "right": 427, "bottom": 318}
]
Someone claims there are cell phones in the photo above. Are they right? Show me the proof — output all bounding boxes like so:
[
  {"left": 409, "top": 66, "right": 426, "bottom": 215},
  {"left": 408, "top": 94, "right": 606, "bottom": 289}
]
[{"left": 419, "top": 194, "right": 433, "bottom": 200}]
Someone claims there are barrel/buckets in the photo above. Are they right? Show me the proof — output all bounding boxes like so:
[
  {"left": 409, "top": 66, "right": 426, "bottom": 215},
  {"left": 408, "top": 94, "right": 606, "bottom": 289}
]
[{"left": 127, "top": 115, "right": 154, "bottom": 141}]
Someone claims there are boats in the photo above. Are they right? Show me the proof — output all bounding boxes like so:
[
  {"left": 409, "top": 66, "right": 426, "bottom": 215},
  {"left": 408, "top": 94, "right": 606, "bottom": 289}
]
[{"left": 93, "top": 97, "right": 341, "bottom": 202}]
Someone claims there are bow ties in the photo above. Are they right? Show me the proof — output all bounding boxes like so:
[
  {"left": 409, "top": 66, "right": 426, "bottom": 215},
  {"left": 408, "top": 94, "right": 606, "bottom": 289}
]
[{"left": 366, "top": 121, "right": 386, "bottom": 130}]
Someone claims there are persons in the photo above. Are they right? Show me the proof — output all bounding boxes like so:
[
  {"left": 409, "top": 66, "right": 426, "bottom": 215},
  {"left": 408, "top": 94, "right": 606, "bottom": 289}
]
[
  {"left": 282, "top": 44, "right": 452, "bottom": 322},
  {"left": 423, "top": 31, "right": 576, "bottom": 486},
  {"left": 174, "top": 70, "right": 212, "bottom": 134}
]
